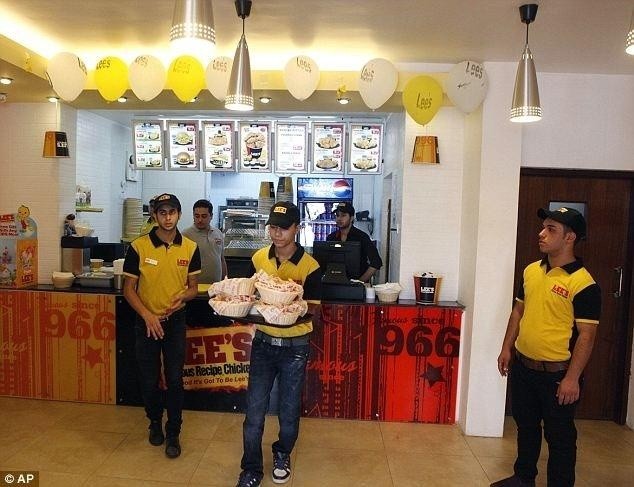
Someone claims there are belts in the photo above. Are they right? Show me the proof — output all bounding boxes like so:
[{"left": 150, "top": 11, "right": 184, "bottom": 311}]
[
  {"left": 514, "top": 350, "right": 570, "bottom": 374},
  {"left": 255, "top": 329, "right": 308, "bottom": 346}
]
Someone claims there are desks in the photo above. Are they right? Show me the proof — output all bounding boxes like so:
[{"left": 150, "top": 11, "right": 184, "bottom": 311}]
[{"left": 0, "top": 283, "right": 467, "bottom": 426}]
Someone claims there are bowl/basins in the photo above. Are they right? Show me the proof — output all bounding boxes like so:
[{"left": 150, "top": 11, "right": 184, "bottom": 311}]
[
  {"left": 376, "top": 292, "right": 398, "bottom": 302},
  {"left": 75, "top": 227, "right": 94, "bottom": 237}
]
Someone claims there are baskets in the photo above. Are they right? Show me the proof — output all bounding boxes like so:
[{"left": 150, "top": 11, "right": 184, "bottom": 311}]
[
  {"left": 91, "top": 260, "right": 103, "bottom": 267},
  {"left": 375, "top": 283, "right": 401, "bottom": 302},
  {"left": 255, "top": 282, "right": 298, "bottom": 305},
  {"left": 52, "top": 274, "right": 74, "bottom": 288},
  {"left": 214, "top": 278, "right": 258, "bottom": 296},
  {"left": 258, "top": 306, "right": 298, "bottom": 326},
  {"left": 210, "top": 300, "right": 253, "bottom": 318}
]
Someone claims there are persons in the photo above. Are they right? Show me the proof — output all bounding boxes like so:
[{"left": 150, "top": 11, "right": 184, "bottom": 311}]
[
  {"left": 490, "top": 207, "right": 601, "bottom": 487},
  {"left": 122, "top": 193, "right": 202, "bottom": 458},
  {"left": 237, "top": 201, "right": 321, "bottom": 487},
  {"left": 139, "top": 195, "right": 158, "bottom": 238},
  {"left": 181, "top": 199, "right": 228, "bottom": 284},
  {"left": 328, "top": 202, "right": 382, "bottom": 283},
  {"left": 308, "top": 203, "right": 337, "bottom": 242}
]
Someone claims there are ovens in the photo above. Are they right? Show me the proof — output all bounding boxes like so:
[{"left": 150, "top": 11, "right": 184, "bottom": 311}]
[{"left": 217, "top": 199, "right": 270, "bottom": 259}]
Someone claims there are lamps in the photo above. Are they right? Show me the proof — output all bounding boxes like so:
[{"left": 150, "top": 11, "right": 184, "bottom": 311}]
[
  {"left": 411, "top": 135, "right": 440, "bottom": 164},
  {"left": 42, "top": 131, "right": 70, "bottom": 158},
  {"left": 508, "top": 4, "right": 543, "bottom": 123},
  {"left": 624, "top": 7, "right": 634, "bottom": 56},
  {"left": 169, "top": 0, "right": 215, "bottom": 55},
  {"left": 225, "top": 0, "right": 255, "bottom": 111}
]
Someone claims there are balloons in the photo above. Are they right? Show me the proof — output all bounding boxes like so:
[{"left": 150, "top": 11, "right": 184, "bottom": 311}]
[
  {"left": 167, "top": 55, "right": 205, "bottom": 102},
  {"left": 92, "top": 56, "right": 129, "bottom": 103},
  {"left": 358, "top": 58, "right": 398, "bottom": 111},
  {"left": 446, "top": 60, "right": 490, "bottom": 118},
  {"left": 45, "top": 52, "right": 86, "bottom": 102},
  {"left": 403, "top": 76, "right": 443, "bottom": 127},
  {"left": 206, "top": 57, "right": 234, "bottom": 101},
  {"left": 283, "top": 55, "right": 320, "bottom": 103},
  {"left": 129, "top": 55, "right": 166, "bottom": 103}
]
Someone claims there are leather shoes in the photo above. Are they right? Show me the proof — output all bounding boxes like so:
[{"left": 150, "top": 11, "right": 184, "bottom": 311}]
[{"left": 490, "top": 473, "right": 535, "bottom": 487}]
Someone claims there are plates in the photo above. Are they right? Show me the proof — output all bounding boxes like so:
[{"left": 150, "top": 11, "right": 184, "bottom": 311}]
[
  {"left": 125, "top": 198, "right": 144, "bottom": 237},
  {"left": 353, "top": 142, "right": 377, "bottom": 149},
  {"left": 355, "top": 160, "right": 376, "bottom": 170},
  {"left": 316, "top": 142, "right": 340, "bottom": 149},
  {"left": 316, "top": 162, "right": 338, "bottom": 169}
]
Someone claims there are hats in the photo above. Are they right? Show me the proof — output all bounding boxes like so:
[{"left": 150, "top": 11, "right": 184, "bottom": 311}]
[
  {"left": 538, "top": 208, "right": 586, "bottom": 239},
  {"left": 265, "top": 201, "right": 300, "bottom": 228},
  {"left": 333, "top": 202, "right": 354, "bottom": 216},
  {"left": 154, "top": 194, "right": 181, "bottom": 211}
]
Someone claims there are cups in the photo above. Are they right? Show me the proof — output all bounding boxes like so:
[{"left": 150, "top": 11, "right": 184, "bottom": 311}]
[
  {"left": 90, "top": 259, "right": 103, "bottom": 272},
  {"left": 365, "top": 288, "right": 377, "bottom": 301},
  {"left": 114, "top": 274, "right": 123, "bottom": 289}
]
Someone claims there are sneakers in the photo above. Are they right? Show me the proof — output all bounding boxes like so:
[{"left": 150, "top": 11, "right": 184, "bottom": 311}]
[
  {"left": 165, "top": 435, "right": 181, "bottom": 458},
  {"left": 236, "top": 471, "right": 264, "bottom": 487},
  {"left": 149, "top": 422, "right": 164, "bottom": 446},
  {"left": 272, "top": 451, "right": 291, "bottom": 484}
]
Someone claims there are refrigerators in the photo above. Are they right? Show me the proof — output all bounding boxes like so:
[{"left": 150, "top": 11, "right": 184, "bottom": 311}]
[{"left": 296, "top": 178, "right": 354, "bottom": 257}]
